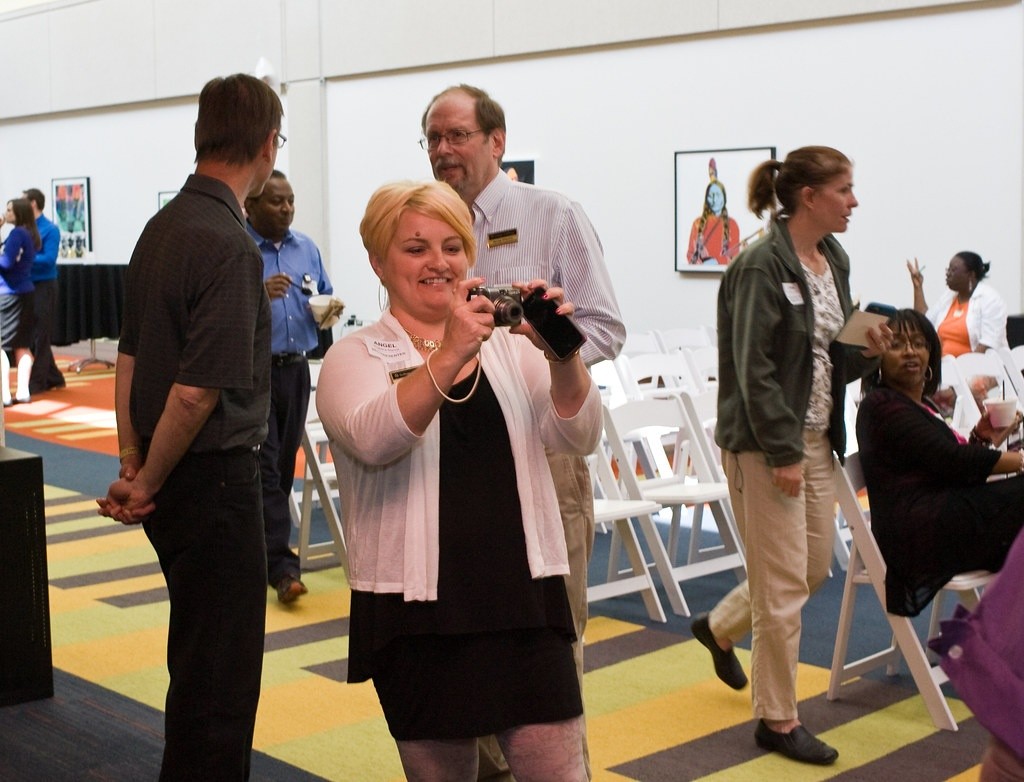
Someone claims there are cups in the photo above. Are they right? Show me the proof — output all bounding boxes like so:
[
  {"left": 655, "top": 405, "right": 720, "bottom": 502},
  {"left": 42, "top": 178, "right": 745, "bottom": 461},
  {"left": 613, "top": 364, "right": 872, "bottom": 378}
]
[
  {"left": 983, "top": 396, "right": 1017, "bottom": 428},
  {"left": 308, "top": 295, "right": 332, "bottom": 326}
]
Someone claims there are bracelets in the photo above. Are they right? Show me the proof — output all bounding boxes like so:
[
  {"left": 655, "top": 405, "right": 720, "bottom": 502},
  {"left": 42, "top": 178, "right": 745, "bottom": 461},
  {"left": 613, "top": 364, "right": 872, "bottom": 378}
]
[
  {"left": 119, "top": 446, "right": 142, "bottom": 465},
  {"left": 544, "top": 348, "right": 579, "bottom": 364}
]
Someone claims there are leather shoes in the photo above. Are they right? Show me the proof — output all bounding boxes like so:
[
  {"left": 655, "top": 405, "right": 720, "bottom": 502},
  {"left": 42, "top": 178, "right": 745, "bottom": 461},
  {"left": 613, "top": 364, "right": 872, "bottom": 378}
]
[
  {"left": 754, "top": 718, "right": 839, "bottom": 766},
  {"left": 688, "top": 611, "right": 748, "bottom": 689}
]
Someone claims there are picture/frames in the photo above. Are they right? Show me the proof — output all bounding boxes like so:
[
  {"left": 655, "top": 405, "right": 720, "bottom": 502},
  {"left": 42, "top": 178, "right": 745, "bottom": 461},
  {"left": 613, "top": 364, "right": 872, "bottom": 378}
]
[
  {"left": 673, "top": 146, "right": 777, "bottom": 273},
  {"left": 51, "top": 175, "right": 91, "bottom": 265}
]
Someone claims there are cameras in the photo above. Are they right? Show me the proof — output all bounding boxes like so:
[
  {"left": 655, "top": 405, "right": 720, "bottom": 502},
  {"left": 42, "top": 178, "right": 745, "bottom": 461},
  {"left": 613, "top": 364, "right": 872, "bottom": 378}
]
[{"left": 468, "top": 285, "right": 524, "bottom": 327}]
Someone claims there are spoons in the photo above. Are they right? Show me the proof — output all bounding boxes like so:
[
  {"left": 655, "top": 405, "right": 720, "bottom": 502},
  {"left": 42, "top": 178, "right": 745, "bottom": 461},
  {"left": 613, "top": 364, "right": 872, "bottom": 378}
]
[{"left": 288, "top": 280, "right": 312, "bottom": 296}]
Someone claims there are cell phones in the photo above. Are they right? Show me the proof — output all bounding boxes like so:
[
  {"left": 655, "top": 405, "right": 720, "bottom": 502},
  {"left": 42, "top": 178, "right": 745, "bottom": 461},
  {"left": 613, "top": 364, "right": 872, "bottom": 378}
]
[{"left": 521, "top": 286, "right": 588, "bottom": 361}]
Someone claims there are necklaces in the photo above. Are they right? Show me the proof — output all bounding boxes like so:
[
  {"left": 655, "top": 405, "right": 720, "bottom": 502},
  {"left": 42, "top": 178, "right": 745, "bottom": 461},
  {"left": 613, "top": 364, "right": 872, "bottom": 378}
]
[{"left": 403, "top": 327, "right": 442, "bottom": 351}]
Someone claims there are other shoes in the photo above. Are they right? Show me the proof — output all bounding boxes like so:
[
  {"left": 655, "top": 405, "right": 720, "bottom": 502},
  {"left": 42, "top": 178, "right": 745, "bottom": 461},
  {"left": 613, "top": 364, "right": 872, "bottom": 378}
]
[
  {"left": 12, "top": 395, "right": 31, "bottom": 403},
  {"left": 277, "top": 573, "right": 309, "bottom": 604},
  {"left": 29, "top": 370, "right": 66, "bottom": 393}
]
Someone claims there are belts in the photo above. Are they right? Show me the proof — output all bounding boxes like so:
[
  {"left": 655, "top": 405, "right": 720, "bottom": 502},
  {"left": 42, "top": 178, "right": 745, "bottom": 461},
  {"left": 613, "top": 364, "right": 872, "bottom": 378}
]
[{"left": 272, "top": 353, "right": 306, "bottom": 366}]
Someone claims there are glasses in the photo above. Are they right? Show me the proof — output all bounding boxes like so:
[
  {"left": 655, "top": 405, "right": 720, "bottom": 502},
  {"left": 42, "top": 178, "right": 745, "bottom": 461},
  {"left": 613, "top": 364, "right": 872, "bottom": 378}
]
[
  {"left": 418, "top": 127, "right": 497, "bottom": 150},
  {"left": 887, "top": 340, "right": 932, "bottom": 353}
]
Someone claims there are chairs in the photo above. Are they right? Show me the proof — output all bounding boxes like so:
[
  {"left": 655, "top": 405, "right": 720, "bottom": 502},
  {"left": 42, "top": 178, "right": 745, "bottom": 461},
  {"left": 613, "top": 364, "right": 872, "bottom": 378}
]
[{"left": 290, "top": 318, "right": 1000, "bottom": 733}]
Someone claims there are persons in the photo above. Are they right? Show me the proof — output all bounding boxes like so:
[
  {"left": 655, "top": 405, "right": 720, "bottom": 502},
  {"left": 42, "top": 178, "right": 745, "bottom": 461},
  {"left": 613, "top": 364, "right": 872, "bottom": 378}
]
[
  {"left": 906, "top": 250, "right": 1010, "bottom": 358},
  {"left": 856, "top": 308, "right": 1024, "bottom": 616},
  {"left": 0, "top": 198, "right": 42, "bottom": 407},
  {"left": 96, "top": 73, "right": 286, "bottom": 782},
  {"left": 316, "top": 179, "right": 602, "bottom": 782},
  {"left": 419, "top": 85, "right": 626, "bottom": 782},
  {"left": 925, "top": 526, "right": 1024, "bottom": 782},
  {"left": 689, "top": 145, "right": 894, "bottom": 766},
  {"left": 22, "top": 188, "right": 65, "bottom": 394},
  {"left": 244, "top": 169, "right": 334, "bottom": 603}
]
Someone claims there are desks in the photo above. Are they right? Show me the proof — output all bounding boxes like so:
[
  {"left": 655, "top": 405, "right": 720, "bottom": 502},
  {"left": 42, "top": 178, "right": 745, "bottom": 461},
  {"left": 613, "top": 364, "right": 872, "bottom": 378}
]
[{"left": 47, "top": 264, "right": 133, "bottom": 372}]
